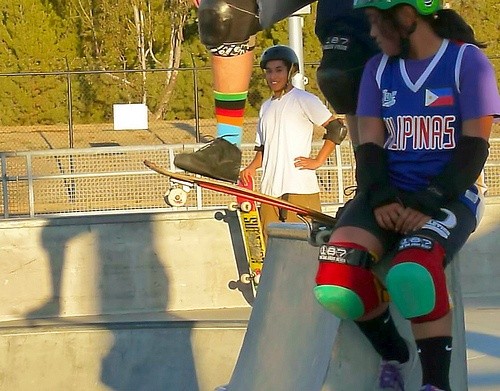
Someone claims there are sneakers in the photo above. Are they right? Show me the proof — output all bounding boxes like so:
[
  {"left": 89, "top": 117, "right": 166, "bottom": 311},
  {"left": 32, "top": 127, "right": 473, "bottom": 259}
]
[
  {"left": 420, "top": 384, "right": 442, "bottom": 390},
  {"left": 377, "top": 337, "right": 415, "bottom": 390},
  {"left": 173, "top": 132, "right": 242, "bottom": 184}
]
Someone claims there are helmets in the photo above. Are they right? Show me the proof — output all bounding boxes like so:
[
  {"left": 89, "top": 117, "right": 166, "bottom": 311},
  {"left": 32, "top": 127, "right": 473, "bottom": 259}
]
[
  {"left": 352, "top": 0, "right": 442, "bottom": 16},
  {"left": 259, "top": 46, "right": 300, "bottom": 73}
]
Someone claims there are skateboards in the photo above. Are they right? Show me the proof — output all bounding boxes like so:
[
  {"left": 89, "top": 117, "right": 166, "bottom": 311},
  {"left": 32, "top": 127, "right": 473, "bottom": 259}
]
[
  {"left": 143, "top": 159, "right": 337, "bottom": 247},
  {"left": 230, "top": 175, "right": 267, "bottom": 298}
]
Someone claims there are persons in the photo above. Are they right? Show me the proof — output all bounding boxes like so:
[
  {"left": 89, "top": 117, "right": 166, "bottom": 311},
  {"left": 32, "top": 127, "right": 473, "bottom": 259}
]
[
  {"left": 237, "top": 43, "right": 347, "bottom": 247},
  {"left": 314, "top": 0, "right": 500, "bottom": 391},
  {"left": 174, "top": 0, "right": 384, "bottom": 183}
]
